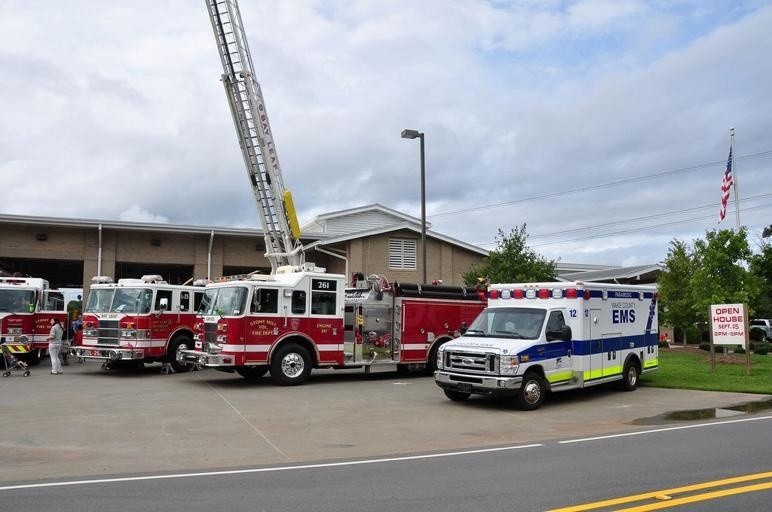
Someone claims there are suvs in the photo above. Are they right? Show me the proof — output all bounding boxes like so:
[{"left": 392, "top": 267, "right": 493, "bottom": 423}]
[{"left": 749, "top": 318, "right": 771, "bottom": 341}]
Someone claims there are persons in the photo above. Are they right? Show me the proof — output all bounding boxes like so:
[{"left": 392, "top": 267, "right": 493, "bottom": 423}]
[
  {"left": 46, "top": 316, "right": 65, "bottom": 375},
  {"left": 660, "top": 333, "right": 668, "bottom": 342},
  {"left": 73, "top": 315, "right": 82, "bottom": 336},
  {"left": 74, "top": 323, "right": 83, "bottom": 363}
]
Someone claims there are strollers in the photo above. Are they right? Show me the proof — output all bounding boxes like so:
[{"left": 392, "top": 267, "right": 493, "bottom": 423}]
[{"left": 0, "top": 343, "right": 30, "bottom": 377}]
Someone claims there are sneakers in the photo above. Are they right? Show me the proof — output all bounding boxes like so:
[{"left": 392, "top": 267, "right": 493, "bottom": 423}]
[{"left": 50, "top": 370, "right": 63, "bottom": 376}]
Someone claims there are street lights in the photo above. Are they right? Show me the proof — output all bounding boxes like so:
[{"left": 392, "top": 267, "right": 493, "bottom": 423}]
[{"left": 400, "top": 129, "right": 428, "bottom": 284}]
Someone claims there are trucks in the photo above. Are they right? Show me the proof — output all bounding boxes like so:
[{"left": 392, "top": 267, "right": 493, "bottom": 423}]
[{"left": 433, "top": 280, "right": 659, "bottom": 410}]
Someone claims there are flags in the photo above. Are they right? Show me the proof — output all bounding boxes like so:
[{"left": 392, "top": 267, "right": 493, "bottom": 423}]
[{"left": 717, "top": 143, "right": 732, "bottom": 224}]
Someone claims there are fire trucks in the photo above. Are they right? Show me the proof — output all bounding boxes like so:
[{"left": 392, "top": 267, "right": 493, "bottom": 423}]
[
  {"left": 70, "top": 275, "right": 216, "bottom": 375},
  {"left": 1, "top": 273, "right": 71, "bottom": 367},
  {"left": 181, "top": 2, "right": 491, "bottom": 381}
]
